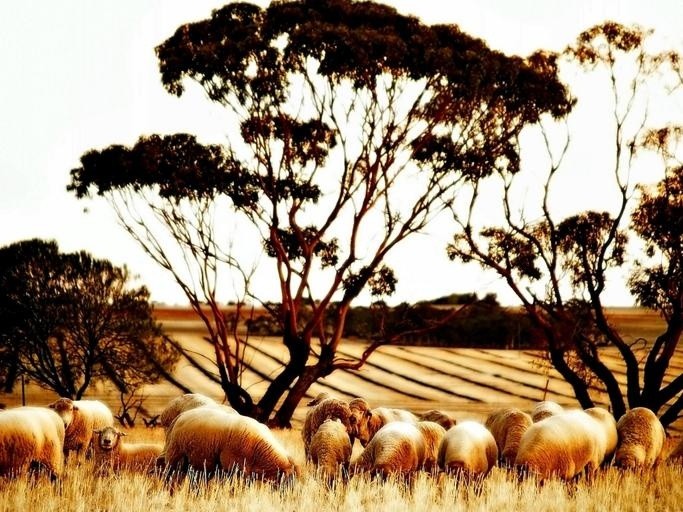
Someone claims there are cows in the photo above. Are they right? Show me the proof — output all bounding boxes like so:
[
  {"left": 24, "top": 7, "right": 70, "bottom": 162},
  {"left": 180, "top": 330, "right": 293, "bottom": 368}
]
[
  {"left": 612, "top": 407, "right": 666, "bottom": 471},
  {"left": 349, "top": 420, "right": 441, "bottom": 492},
  {"left": 412, "top": 410, "right": 459, "bottom": 429},
  {"left": 529, "top": 400, "right": 567, "bottom": 423},
  {"left": 436, "top": 419, "right": 499, "bottom": 496},
  {"left": 484, "top": 407, "right": 535, "bottom": 469},
  {"left": 517, "top": 410, "right": 607, "bottom": 490},
  {"left": 585, "top": 407, "right": 619, "bottom": 460},
  {"left": 306, "top": 392, "right": 334, "bottom": 407},
  {"left": 347, "top": 398, "right": 417, "bottom": 450},
  {"left": 300, "top": 398, "right": 357, "bottom": 488}
]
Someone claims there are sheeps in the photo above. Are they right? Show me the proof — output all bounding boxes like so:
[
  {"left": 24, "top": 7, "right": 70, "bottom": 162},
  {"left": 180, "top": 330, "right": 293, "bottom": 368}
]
[
  {"left": 164, "top": 403, "right": 306, "bottom": 497},
  {"left": 0, "top": 406, "right": 66, "bottom": 495},
  {"left": 47, "top": 398, "right": 115, "bottom": 468},
  {"left": 91, "top": 426, "right": 163, "bottom": 478},
  {"left": 160, "top": 393, "right": 217, "bottom": 434}
]
